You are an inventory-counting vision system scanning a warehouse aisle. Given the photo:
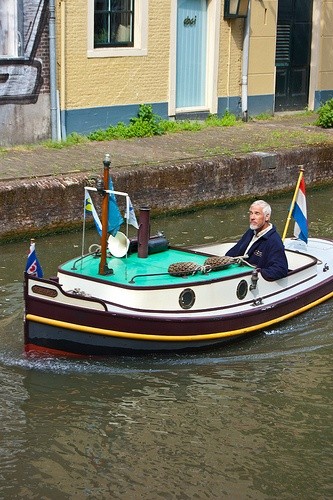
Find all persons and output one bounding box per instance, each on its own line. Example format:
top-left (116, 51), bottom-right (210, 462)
top-left (225, 200), bottom-right (288, 282)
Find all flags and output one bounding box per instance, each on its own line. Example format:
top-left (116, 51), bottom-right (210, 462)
top-left (108, 176), bottom-right (124, 237)
top-left (25, 242), bottom-right (44, 278)
top-left (85, 191), bottom-right (102, 238)
top-left (288, 174), bottom-right (308, 244)
top-left (124, 196), bottom-right (139, 229)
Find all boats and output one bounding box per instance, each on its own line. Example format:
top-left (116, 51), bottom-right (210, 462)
top-left (23, 154), bottom-right (333, 360)
